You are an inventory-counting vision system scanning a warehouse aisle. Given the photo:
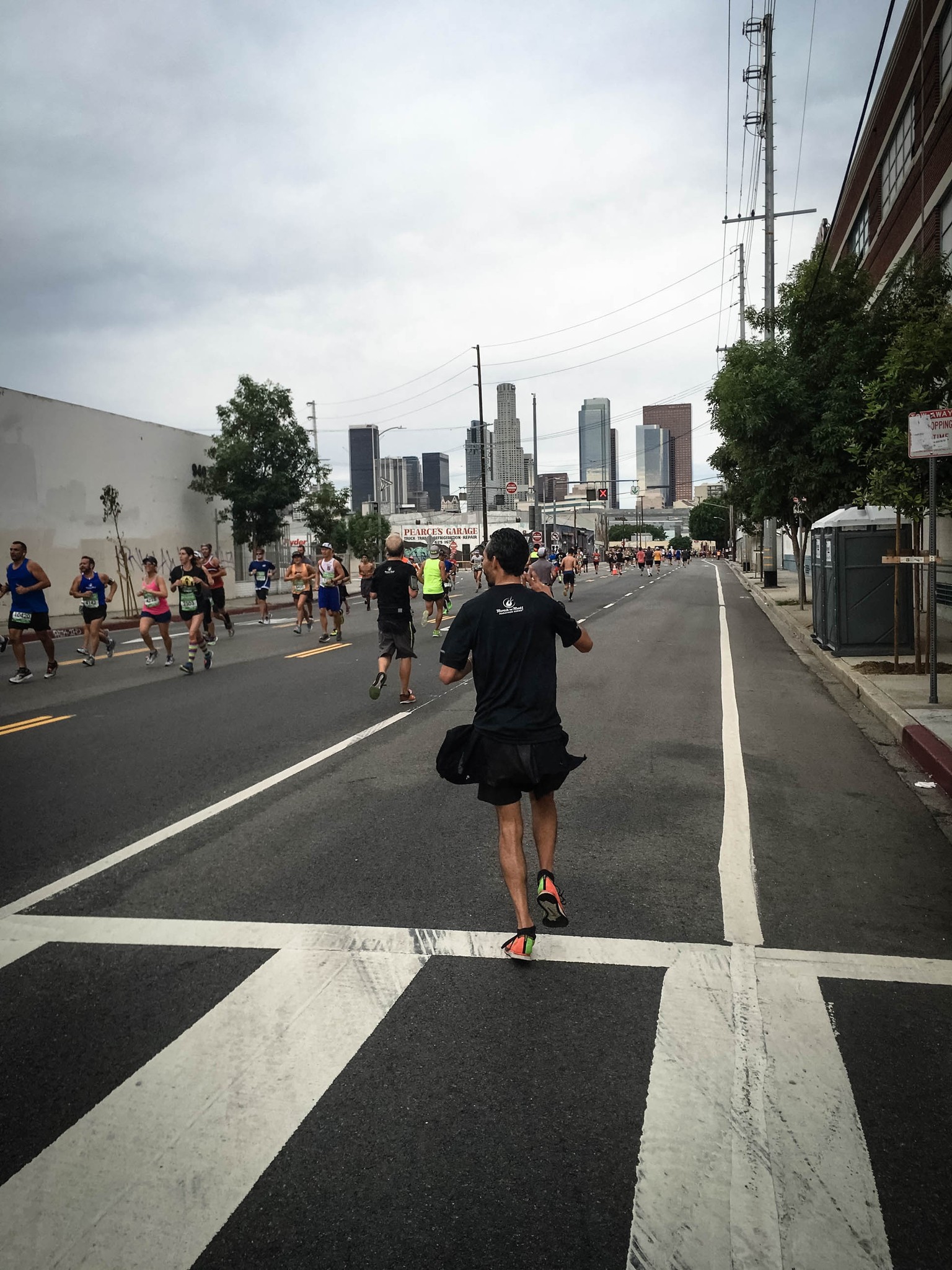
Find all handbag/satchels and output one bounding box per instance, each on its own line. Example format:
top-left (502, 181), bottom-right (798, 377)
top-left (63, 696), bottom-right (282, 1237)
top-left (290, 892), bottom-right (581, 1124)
top-left (436, 724), bottom-right (479, 784)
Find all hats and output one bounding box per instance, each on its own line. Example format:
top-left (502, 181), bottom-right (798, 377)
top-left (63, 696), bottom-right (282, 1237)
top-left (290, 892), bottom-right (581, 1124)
top-left (193, 551), bottom-right (201, 558)
top-left (474, 549), bottom-right (480, 554)
top-left (143, 555), bottom-right (157, 567)
top-left (551, 550), bottom-right (555, 551)
top-left (408, 557), bottom-right (415, 561)
top-left (537, 547), bottom-right (548, 557)
top-left (430, 545), bottom-right (446, 559)
top-left (655, 547), bottom-right (659, 550)
top-left (450, 553), bottom-right (454, 556)
top-left (292, 551), bottom-right (302, 559)
top-left (671, 546), bottom-right (673, 549)
top-left (320, 542), bottom-right (332, 550)
top-left (558, 548), bottom-right (562, 551)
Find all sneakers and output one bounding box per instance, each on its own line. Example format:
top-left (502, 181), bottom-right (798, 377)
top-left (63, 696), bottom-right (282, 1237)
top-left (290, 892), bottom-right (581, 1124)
top-left (307, 615), bottom-right (314, 632)
top-left (447, 598), bottom-right (452, 610)
top-left (329, 629), bottom-right (338, 636)
top-left (258, 618), bottom-right (264, 623)
top-left (365, 598), bottom-right (367, 604)
top-left (77, 648), bottom-right (90, 655)
top-left (228, 627), bottom-right (235, 638)
top-left (569, 598), bottom-right (573, 602)
top-left (610, 563), bottom-right (635, 577)
top-left (504, 934), bottom-right (535, 961)
top-left (563, 586), bottom-right (567, 596)
top-left (165, 653), bottom-right (175, 666)
top-left (104, 629), bottom-right (116, 658)
top-left (400, 689), bottom-right (416, 704)
top-left (663, 560), bottom-right (692, 568)
top-left (146, 648), bottom-right (159, 665)
top-left (82, 655), bottom-right (95, 666)
top-left (369, 672), bottom-right (387, 700)
top-left (179, 662), bottom-right (193, 674)
top-left (264, 613), bottom-right (272, 625)
top-left (0, 637), bottom-right (9, 653)
top-left (202, 631), bottom-right (218, 646)
top-left (640, 567), bottom-right (660, 577)
top-left (294, 625), bottom-right (302, 634)
top-left (596, 572), bottom-right (598, 574)
top-left (578, 570), bottom-right (588, 576)
top-left (421, 610), bottom-right (429, 627)
top-left (559, 580), bottom-right (562, 584)
top-left (442, 608), bottom-right (448, 615)
top-left (476, 589), bottom-right (478, 593)
top-left (556, 572), bottom-right (558, 577)
top-left (536, 875), bottom-right (569, 927)
top-left (432, 630), bottom-right (443, 637)
top-left (336, 630), bottom-right (342, 642)
top-left (479, 584), bottom-right (482, 589)
top-left (9, 667), bottom-right (33, 683)
top-left (319, 633), bottom-right (331, 643)
top-left (367, 606), bottom-right (370, 611)
top-left (339, 609), bottom-right (344, 624)
top-left (346, 605), bottom-right (350, 615)
top-left (204, 651), bottom-right (214, 669)
top-left (43, 660), bottom-right (58, 678)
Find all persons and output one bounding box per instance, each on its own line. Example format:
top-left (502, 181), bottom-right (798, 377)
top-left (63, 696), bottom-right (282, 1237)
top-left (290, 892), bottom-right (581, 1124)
top-left (437, 526), bottom-right (594, 961)
top-left (135, 555), bottom-right (176, 667)
top-left (0, 542), bottom-right (60, 685)
top-left (283, 552), bottom-right (317, 633)
top-left (192, 551), bottom-right (218, 645)
top-left (69, 556), bottom-right (118, 665)
top-left (331, 546), bottom-right (721, 638)
top-left (200, 543), bottom-right (235, 636)
top-left (367, 531), bottom-right (420, 704)
top-left (297, 544), bottom-right (316, 620)
top-left (315, 541), bottom-right (347, 645)
top-left (75, 560), bottom-right (111, 656)
top-left (248, 548), bottom-right (277, 624)
top-left (168, 547), bottom-right (214, 675)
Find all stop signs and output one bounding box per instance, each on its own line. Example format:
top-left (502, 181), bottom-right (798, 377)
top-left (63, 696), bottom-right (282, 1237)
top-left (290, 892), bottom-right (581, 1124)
top-left (505, 481), bottom-right (517, 494)
top-left (532, 532), bottom-right (542, 541)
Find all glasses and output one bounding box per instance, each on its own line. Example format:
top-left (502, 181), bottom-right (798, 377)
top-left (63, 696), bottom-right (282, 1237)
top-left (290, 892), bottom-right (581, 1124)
top-left (595, 550), bottom-right (598, 551)
top-left (256, 553), bottom-right (263, 555)
